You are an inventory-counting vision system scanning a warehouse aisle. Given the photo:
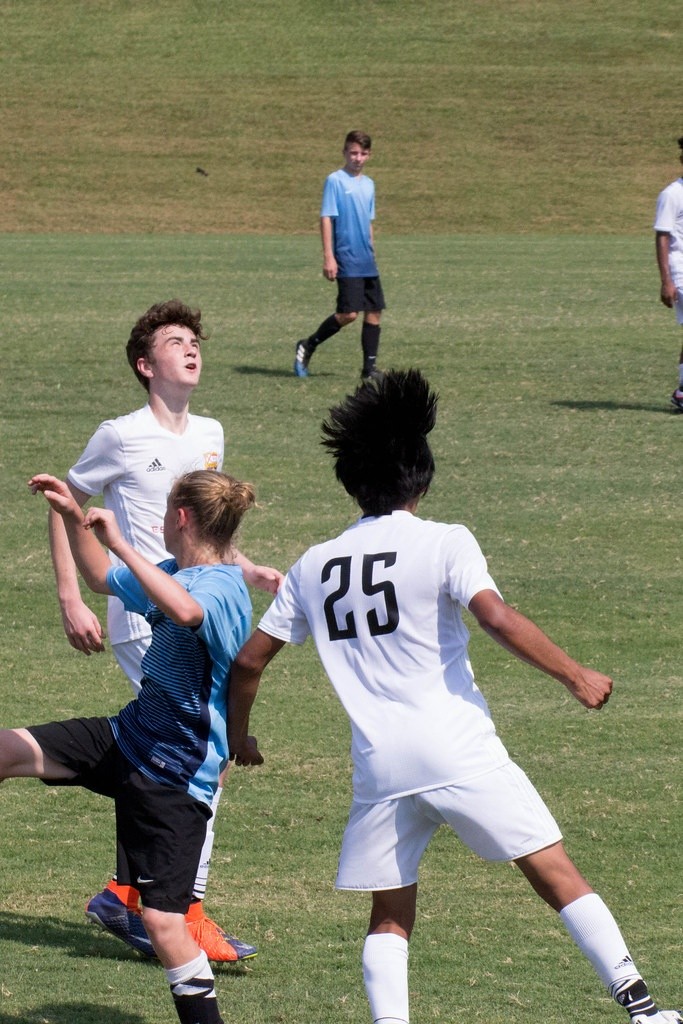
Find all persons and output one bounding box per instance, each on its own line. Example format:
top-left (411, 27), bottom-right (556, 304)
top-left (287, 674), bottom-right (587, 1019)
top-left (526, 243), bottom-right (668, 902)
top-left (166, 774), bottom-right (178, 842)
top-left (294, 130), bottom-right (387, 378)
top-left (47, 298), bottom-right (281, 967)
top-left (0, 471), bottom-right (257, 1024)
top-left (224, 369), bottom-right (683, 1024)
top-left (654, 134), bottom-right (683, 408)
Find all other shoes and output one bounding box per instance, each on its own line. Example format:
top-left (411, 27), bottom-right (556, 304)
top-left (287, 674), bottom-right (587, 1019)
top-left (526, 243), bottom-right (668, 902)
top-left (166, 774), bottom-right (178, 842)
top-left (631, 1010), bottom-right (683, 1024)
top-left (669, 387), bottom-right (683, 407)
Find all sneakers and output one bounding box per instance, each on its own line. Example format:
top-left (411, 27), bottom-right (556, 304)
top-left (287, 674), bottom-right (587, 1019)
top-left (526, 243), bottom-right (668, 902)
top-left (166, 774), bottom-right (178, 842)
top-left (361, 368), bottom-right (385, 382)
top-left (85, 888), bottom-right (158, 958)
top-left (184, 915), bottom-right (258, 967)
top-left (294, 339), bottom-right (315, 377)
